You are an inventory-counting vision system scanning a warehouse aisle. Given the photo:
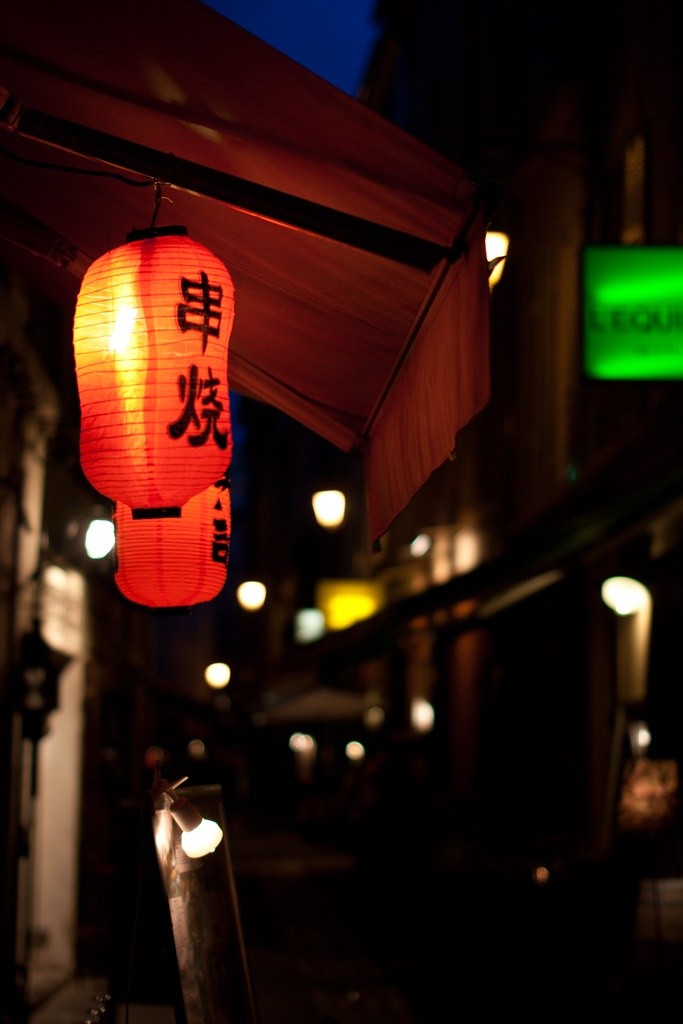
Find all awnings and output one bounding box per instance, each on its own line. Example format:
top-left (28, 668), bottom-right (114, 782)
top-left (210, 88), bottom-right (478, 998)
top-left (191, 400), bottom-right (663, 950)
top-left (0, 0), bottom-right (493, 554)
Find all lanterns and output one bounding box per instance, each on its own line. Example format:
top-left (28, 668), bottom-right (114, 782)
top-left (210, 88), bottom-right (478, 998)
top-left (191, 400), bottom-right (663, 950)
top-left (73, 225), bottom-right (233, 520)
top-left (111, 474), bottom-right (232, 608)
